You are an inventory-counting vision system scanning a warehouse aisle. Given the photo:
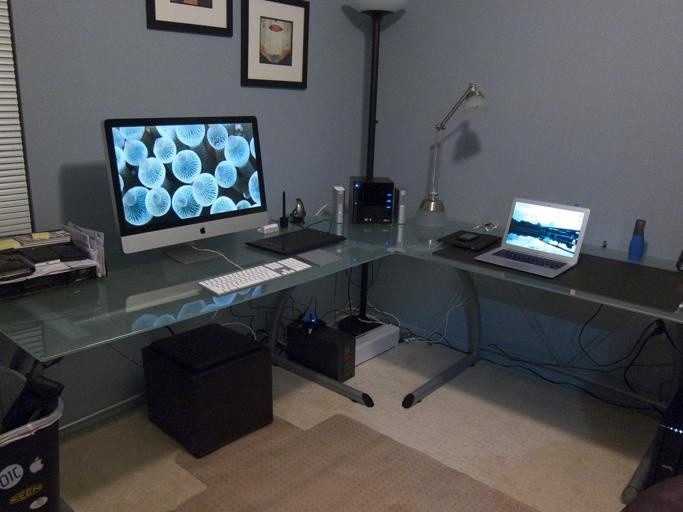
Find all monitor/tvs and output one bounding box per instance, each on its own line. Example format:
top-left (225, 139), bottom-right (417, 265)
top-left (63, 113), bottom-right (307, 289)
top-left (100, 115), bottom-right (269, 265)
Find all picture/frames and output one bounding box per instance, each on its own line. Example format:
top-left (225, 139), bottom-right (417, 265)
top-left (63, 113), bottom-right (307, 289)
top-left (145, 0), bottom-right (233, 37)
top-left (241, 0), bottom-right (309, 90)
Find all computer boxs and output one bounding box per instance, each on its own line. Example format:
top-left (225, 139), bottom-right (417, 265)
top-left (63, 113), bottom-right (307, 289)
top-left (286, 317), bottom-right (355, 383)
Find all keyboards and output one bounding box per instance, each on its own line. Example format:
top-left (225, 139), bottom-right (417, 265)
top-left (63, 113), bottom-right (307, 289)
top-left (196, 257), bottom-right (312, 296)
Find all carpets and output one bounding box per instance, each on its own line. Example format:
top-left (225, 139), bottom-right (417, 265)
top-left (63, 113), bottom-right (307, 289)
top-left (169, 413), bottom-right (539, 512)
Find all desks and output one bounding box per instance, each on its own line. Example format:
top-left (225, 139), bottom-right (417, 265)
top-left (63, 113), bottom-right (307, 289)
top-left (1, 218), bottom-right (683, 512)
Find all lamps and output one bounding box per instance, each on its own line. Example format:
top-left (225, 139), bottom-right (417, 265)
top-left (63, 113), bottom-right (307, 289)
top-left (411, 82), bottom-right (492, 227)
top-left (342, 2), bottom-right (407, 317)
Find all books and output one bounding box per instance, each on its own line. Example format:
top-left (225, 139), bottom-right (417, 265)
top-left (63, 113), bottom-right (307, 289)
top-left (0, 219), bottom-right (108, 280)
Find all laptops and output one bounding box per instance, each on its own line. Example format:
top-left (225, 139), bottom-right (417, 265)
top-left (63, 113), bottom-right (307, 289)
top-left (474, 197), bottom-right (592, 279)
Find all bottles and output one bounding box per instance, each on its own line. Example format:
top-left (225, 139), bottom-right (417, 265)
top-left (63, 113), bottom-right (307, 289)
top-left (627, 218), bottom-right (646, 259)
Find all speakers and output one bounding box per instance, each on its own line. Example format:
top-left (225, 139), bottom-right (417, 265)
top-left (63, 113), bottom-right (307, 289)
top-left (350, 176), bottom-right (396, 224)
